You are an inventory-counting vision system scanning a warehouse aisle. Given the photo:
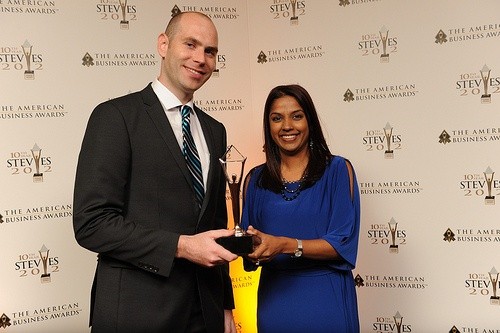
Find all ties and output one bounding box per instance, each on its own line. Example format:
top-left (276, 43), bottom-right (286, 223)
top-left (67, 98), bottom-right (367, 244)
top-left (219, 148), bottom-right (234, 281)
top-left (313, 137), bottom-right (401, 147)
top-left (178, 105), bottom-right (205, 213)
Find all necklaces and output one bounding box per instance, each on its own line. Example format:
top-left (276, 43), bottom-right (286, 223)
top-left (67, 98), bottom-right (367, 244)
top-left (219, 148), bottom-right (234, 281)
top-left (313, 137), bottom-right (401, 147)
top-left (279, 164), bottom-right (308, 200)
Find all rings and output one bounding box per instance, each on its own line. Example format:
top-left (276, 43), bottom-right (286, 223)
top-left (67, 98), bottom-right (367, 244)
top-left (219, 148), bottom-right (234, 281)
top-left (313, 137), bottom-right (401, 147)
top-left (256, 259), bottom-right (260, 266)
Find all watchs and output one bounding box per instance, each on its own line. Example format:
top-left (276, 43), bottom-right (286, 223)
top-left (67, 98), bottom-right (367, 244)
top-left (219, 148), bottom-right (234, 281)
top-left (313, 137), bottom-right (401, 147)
top-left (291, 238), bottom-right (303, 259)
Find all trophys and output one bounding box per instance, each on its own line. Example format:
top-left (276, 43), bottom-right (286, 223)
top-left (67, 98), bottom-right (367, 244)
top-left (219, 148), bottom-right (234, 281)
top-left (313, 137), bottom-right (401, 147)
top-left (219, 144), bottom-right (260, 255)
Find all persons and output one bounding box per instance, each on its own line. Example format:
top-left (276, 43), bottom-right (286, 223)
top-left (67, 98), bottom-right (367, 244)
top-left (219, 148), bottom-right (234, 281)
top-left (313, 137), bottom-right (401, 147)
top-left (73, 12), bottom-right (239, 333)
top-left (237, 85), bottom-right (361, 333)
